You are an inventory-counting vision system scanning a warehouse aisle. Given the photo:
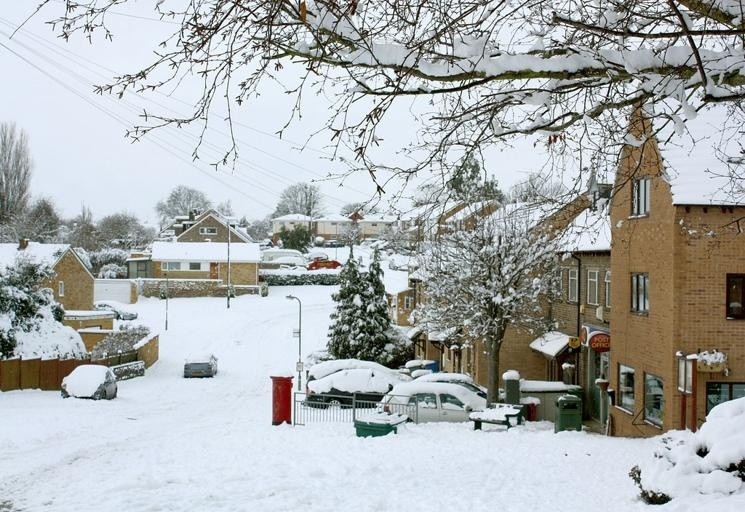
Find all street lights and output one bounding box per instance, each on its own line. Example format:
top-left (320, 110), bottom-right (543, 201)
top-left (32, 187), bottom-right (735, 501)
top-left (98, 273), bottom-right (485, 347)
top-left (287, 294), bottom-right (303, 392)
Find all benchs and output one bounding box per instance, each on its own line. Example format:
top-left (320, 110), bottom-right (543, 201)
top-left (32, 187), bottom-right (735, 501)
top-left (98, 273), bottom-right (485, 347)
top-left (468, 403), bottom-right (524, 432)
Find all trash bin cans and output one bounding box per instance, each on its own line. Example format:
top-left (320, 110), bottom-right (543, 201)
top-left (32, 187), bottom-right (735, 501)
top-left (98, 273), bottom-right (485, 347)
top-left (354, 414), bottom-right (408, 438)
top-left (556, 395), bottom-right (583, 433)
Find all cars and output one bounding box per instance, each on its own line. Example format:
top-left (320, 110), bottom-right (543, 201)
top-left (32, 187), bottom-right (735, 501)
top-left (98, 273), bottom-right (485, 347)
top-left (61, 364), bottom-right (118, 399)
top-left (94, 300), bottom-right (138, 320)
top-left (306, 358), bottom-right (487, 422)
top-left (185, 353), bottom-right (218, 378)
